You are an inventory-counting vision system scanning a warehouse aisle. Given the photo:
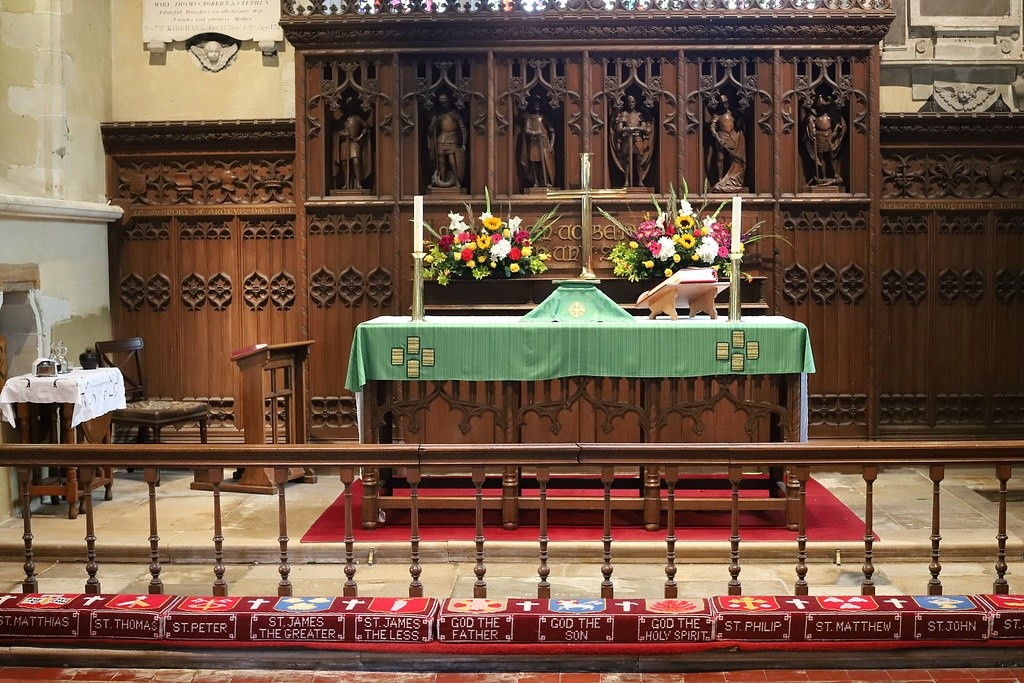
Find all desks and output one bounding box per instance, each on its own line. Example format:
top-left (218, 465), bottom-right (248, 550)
top-left (0, 366), bottom-right (128, 517)
top-left (343, 315), bottom-right (818, 532)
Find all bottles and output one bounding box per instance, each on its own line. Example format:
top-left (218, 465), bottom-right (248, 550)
top-left (47, 340), bottom-right (68, 372)
top-left (78, 346), bottom-right (99, 369)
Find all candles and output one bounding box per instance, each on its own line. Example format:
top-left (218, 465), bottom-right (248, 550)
top-left (413, 195), bottom-right (424, 253)
top-left (730, 193), bottom-right (742, 254)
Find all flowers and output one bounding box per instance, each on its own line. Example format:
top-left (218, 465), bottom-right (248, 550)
top-left (595, 177), bottom-right (797, 284)
top-left (407, 186), bottom-right (563, 286)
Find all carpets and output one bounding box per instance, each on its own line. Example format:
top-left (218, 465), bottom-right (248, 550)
top-left (299, 471), bottom-right (882, 544)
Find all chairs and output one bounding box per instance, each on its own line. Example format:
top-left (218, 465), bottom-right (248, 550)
top-left (94, 336), bottom-right (211, 487)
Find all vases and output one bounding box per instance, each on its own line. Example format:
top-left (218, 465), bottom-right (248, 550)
top-left (646, 275), bottom-right (768, 304)
top-left (531, 277), bottom-right (650, 304)
top-left (423, 277), bottom-right (531, 305)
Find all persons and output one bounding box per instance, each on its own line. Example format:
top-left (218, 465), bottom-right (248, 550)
top-left (805, 92), bottom-right (846, 186)
top-left (428, 93), bottom-right (467, 186)
top-left (956, 83), bottom-right (973, 102)
top-left (615, 95), bottom-right (652, 186)
top-left (525, 93), bottom-right (557, 188)
top-left (708, 94), bottom-right (747, 192)
top-left (332, 95), bottom-right (372, 189)
